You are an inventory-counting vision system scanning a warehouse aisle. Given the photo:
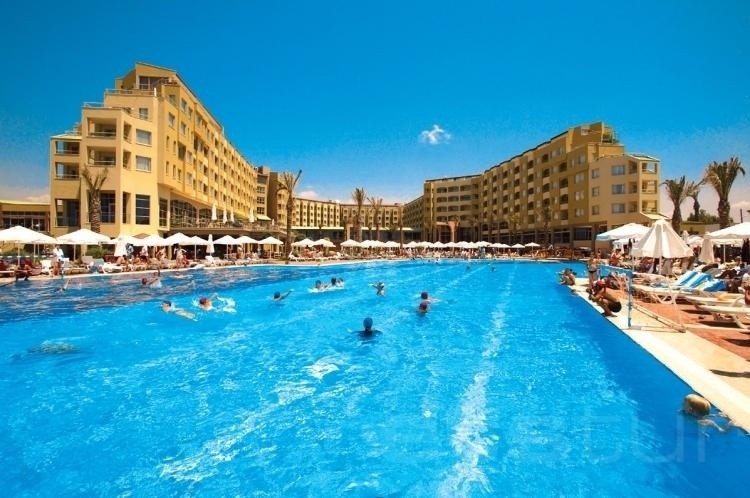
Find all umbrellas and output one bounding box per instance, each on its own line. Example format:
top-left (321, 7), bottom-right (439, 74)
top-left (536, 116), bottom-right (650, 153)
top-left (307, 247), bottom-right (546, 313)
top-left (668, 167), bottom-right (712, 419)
top-left (698, 227), bottom-right (715, 267)
top-left (595, 221), bottom-right (653, 240)
top-left (630, 216), bottom-right (698, 276)
top-left (708, 221), bottom-right (750, 244)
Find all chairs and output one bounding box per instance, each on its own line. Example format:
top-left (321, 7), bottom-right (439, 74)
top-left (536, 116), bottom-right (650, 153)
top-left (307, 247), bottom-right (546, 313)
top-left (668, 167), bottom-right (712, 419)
top-left (631, 271), bottom-right (750, 329)
top-left (182, 253), bottom-right (349, 267)
top-left (40, 253), bottom-right (188, 277)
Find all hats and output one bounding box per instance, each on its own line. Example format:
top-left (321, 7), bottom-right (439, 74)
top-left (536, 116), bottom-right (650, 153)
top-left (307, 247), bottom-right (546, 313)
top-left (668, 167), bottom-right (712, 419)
top-left (163, 299), bottom-right (171, 306)
top-left (364, 317), bottom-right (373, 327)
top-left (595, 279), bottom-right (606, 287)
top-left (316, 277), bottom-right (322, 284)
top-left (421, 291), bottom-right (427, 298)
top-left (274, 292), bottom-right (280, 298)
top-left (378, 282), bottom-right (385, 288)
top-left (200, 298), bottom-right (206, 304)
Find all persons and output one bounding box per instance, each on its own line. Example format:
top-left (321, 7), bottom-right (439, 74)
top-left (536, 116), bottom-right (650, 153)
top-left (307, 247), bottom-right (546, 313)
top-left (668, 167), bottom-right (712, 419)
top-left (569, 288), bottom-right (582, 295)
top-left (193, 290), bottom-right (219, 311)
top-left (271, 288), bottom-right (294, 302)
top-left (586, 237), bottom-right (750, 318)
top-left (555, 269), bottom-right (573, 280)
top-left (416, 300), bottom-right (429, 320)
top-left (421, 290), bottom-right (439, 305)
top-left (372, 281), bottom-right (385, 297)
top-left (159, 299), bottom-right (194, 320)
top-left (0, 244), bottom-right (196, 289)
top-left (330, 276), bottom-right (344, 287)
top-left (348, 314), bottom-right (385, 347)
top-left (677, 391), bottom-right (738, 439)
top-left (315, 280), bottom-right (328, 290)
top-left (560, 267), bottom-right (577, 286)
